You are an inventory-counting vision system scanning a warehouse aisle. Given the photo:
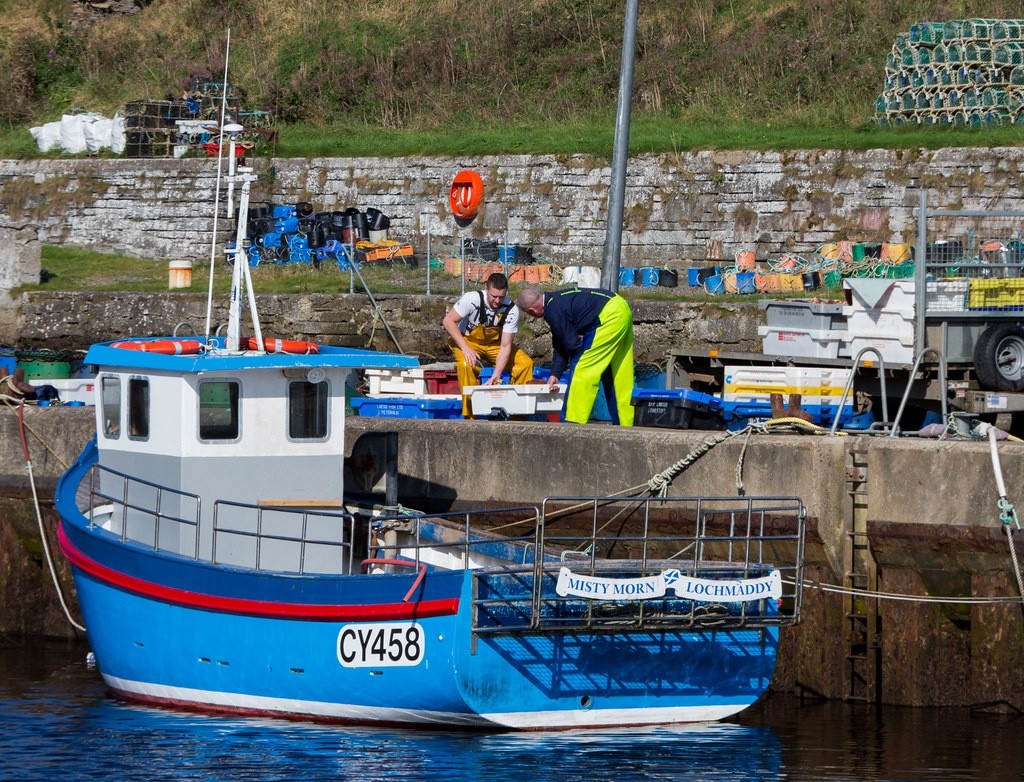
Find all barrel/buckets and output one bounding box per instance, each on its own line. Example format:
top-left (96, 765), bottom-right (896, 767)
top-left (226, 202), bottom-right (391, 272)
top-left (446, 238), bottom-right (1024, 293)
top-left (169, 260), bottom-right (193, 289)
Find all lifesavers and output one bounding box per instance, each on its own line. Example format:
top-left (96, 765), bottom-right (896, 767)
top-left (224, 335), bottom-right (321, 355)
top-left (109, 338), bottom-right (203, 356)
top-left (459, 184), bottom-right (474, 208)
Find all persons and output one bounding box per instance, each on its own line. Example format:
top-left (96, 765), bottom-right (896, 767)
top-left (444, 275), bottom-right (534, 418)
top-left (516, 288), bottom-right (638, 427)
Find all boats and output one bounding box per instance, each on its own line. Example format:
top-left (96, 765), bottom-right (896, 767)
top-left (16, 122), bottom-right (807, 733)
